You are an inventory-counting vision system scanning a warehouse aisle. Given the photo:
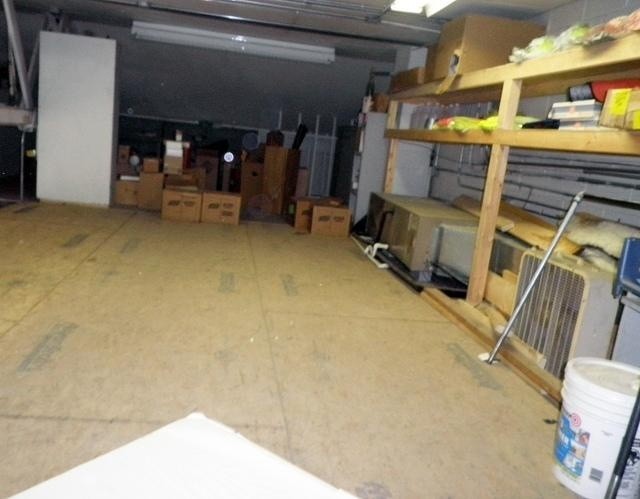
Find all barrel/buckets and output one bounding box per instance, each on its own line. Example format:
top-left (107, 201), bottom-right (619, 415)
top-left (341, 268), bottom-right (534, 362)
top-left (552, 358), bottom-right (640, 499)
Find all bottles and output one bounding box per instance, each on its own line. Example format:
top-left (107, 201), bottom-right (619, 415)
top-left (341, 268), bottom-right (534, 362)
top-left (410, 101), bottom-right (493, 132)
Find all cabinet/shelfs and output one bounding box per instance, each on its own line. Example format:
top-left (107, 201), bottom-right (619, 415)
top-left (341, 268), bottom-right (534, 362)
top-left (364, 31), bottom-right (640, 410)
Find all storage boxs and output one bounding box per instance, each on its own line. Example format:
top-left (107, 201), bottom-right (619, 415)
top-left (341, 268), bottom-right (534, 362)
top-left (114, 168), bottom-right (243, 229)
top-left (392, 13), bottom-right (545, 92)
top-left (283, 196), bottom-right (354, 238)
